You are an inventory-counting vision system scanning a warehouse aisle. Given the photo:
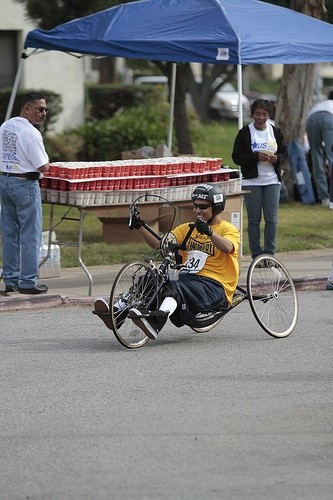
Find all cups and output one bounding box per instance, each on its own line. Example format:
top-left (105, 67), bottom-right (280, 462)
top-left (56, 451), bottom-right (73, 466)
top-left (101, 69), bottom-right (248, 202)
top-left (39, 155), bottom-right (242, 206)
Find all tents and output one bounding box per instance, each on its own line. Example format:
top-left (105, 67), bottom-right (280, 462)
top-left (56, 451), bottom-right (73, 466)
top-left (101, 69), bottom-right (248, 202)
top-left (5, 0), bottom-right (333, 157)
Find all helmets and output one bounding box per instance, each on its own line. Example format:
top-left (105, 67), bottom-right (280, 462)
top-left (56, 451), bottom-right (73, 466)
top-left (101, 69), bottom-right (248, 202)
top-left (192, 183), bottom-right (225, 214)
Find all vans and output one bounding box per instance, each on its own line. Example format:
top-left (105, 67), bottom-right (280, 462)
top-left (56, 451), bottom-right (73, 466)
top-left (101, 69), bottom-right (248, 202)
top-left (134, 76), bottom-right (252, 121)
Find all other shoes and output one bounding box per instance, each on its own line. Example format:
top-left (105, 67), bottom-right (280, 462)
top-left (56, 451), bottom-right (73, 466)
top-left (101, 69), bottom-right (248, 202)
top-left (322, 200), bottom-right (333, 209)
top-left (326, 280), bottom-right (333, 290)
top-left (255, 260), bottom-right (280, 269)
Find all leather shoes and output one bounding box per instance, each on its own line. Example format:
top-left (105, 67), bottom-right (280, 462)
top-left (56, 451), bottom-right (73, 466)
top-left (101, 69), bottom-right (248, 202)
top-left (20, 284), bottom-right (48, 295)
top-left (6, 285), bottom-right (19, 292)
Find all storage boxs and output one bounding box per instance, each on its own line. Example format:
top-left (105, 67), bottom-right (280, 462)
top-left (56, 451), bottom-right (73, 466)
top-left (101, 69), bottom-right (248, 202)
top-left (93, 194), bottom-right (242, 260)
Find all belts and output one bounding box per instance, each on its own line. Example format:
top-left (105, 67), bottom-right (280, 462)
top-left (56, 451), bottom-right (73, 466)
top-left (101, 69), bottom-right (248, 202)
top-left (4, 172), bottom-right (27, 178)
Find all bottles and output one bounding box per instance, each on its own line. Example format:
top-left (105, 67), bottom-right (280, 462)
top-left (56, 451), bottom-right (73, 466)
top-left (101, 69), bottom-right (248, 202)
top-left (38, 230), bottom-right (61, 278)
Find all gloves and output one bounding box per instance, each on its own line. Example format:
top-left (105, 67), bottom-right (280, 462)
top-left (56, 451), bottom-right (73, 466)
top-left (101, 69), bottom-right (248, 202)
top-left (196, 219), bottom-right (212, 236)
top-left (129, 209), bottom-right (142, 230)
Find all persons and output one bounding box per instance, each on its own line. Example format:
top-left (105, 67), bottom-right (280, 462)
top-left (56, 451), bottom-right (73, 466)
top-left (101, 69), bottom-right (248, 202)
top-left (231, 99), bottom-right (288, 268)
top-left (94, 183), bottom-right (242, 340)
top-left (0, 91), bottom-right (51, 293)
top-left (305, 91), bottom-right (333, 209)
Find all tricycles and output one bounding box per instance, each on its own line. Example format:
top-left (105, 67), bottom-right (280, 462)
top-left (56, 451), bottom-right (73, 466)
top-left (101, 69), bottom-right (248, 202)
top-left (91, 194), bottom-right (300, 349)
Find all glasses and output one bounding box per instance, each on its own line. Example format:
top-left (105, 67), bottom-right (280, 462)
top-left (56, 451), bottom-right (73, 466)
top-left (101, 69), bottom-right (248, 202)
top-left (27, 103), bottom-right (49, 113)
top-left (194, 203), bottom-right (211, 210)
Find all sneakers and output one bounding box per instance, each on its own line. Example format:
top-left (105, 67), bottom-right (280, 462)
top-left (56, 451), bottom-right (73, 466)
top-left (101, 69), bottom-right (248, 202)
top-left (94, 297), bottom-right (123, 331)
top-left (128, 308), bottom-right (159, 340)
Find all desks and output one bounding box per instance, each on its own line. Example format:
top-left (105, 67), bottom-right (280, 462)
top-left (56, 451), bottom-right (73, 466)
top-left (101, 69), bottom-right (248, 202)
top-left (38, 190), bottom-right (251, 296)
